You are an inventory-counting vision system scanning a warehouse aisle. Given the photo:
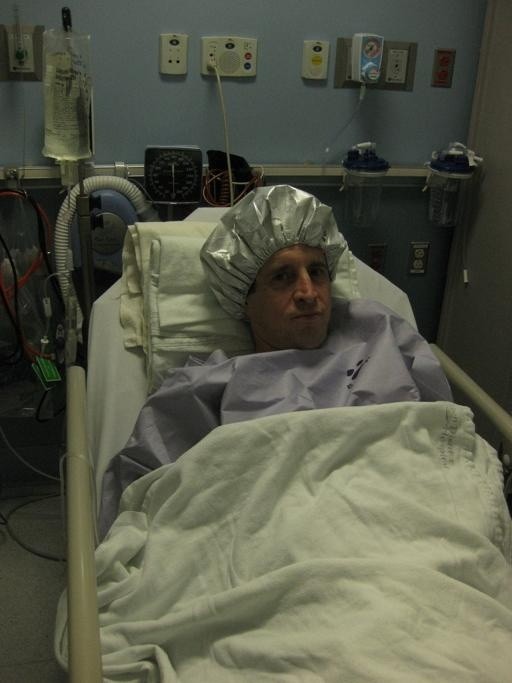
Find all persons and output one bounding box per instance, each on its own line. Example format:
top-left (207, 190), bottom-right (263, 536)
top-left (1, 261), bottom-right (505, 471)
top-left (96, 178), bottom-right (454, 539)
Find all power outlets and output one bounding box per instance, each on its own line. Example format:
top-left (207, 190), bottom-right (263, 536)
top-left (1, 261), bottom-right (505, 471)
top-left (410, 242), bottom-right (429, 274)
top-left (200, 38), bottom-right (258, 77)
top-left (8, 32), bottom-right (34, 73)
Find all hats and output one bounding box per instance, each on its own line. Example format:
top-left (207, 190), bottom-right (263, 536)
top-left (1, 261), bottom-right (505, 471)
top-left (200, 185), bottom-right (349, 322)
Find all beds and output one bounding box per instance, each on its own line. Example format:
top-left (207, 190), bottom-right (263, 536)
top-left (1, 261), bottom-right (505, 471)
top-left (65, 205), bottom-right (512, 682)
